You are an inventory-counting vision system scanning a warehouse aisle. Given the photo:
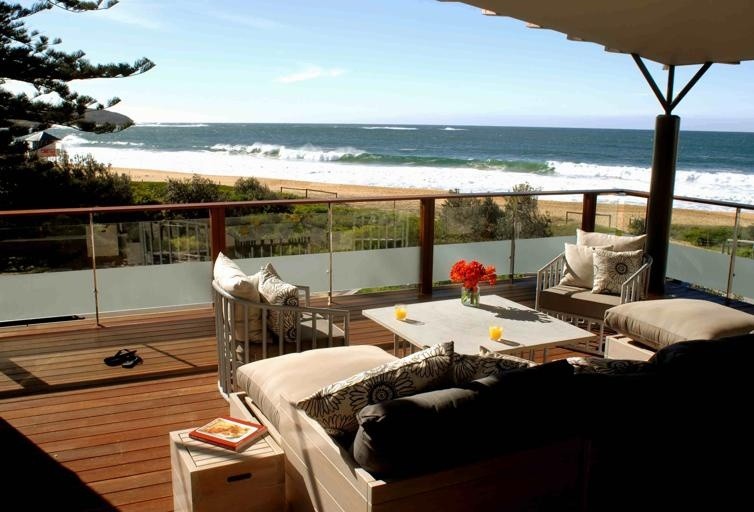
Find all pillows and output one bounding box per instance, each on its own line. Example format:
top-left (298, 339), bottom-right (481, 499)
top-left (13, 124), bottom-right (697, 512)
top-left (349, 359), bottom-right (575, 473)
top-left (592, 247), bottom-right (643, 298)
top-left (575, 227), bottom-right (649, 297)
top-left (213, 251), bottom-right (272, 344)
top-left (648, 333), bottom-right (754, 403)
top-left (603, 298), bottom-right (754, 350)
top-left (456, 352), bottom-right (530, 383)
top-left (294, 342), bottom-right (455, 479)
top-left (572, 356), bottom-right (664, 397)
top-left (479, 344), bottom-right (539, 369)
top-left (257, 263), bottom-right (298, 344)
top-left (559, 243), bottom-right (614, 291)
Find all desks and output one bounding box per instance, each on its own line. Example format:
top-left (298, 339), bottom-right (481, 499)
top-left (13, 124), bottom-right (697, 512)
top-left (361, 294), bottom-right (598, 363)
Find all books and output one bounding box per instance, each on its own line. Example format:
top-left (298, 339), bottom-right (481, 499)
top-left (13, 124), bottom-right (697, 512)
top-left (188, 414), bottom-right (269, 453)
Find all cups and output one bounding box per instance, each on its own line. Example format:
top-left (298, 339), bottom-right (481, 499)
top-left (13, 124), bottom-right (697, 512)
top-left (489, 319), bottom-right (504, 342)
top-left (395, 305), bottom-right (408, 322)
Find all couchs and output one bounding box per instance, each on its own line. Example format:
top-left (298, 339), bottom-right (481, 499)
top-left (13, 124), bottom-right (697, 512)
top-left (229, 297), bottom-right (754, 512)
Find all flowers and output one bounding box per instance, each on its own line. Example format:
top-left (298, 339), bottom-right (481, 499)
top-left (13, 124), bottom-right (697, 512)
top-left (449, 258), bottom-right (497, 308)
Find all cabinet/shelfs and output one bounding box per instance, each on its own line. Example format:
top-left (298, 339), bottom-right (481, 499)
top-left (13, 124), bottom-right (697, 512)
top-left (169, 416), bottom-right (286, 512)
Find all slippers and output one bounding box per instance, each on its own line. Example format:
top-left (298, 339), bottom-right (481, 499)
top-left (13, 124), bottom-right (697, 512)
top-left (104, 349), bottom-right (136, 366)
top-left (122, 356), bottom-right (141, 368)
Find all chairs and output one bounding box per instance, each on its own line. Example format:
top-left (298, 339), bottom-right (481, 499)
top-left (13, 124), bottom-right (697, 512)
top-left (535, 242), bottom-right (654, 338)
top-left (211, 279), bottom-right (351, 401)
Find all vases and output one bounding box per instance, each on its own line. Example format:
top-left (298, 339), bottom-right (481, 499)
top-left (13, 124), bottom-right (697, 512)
top-left (460, 286), bottom-right (481, 308)
